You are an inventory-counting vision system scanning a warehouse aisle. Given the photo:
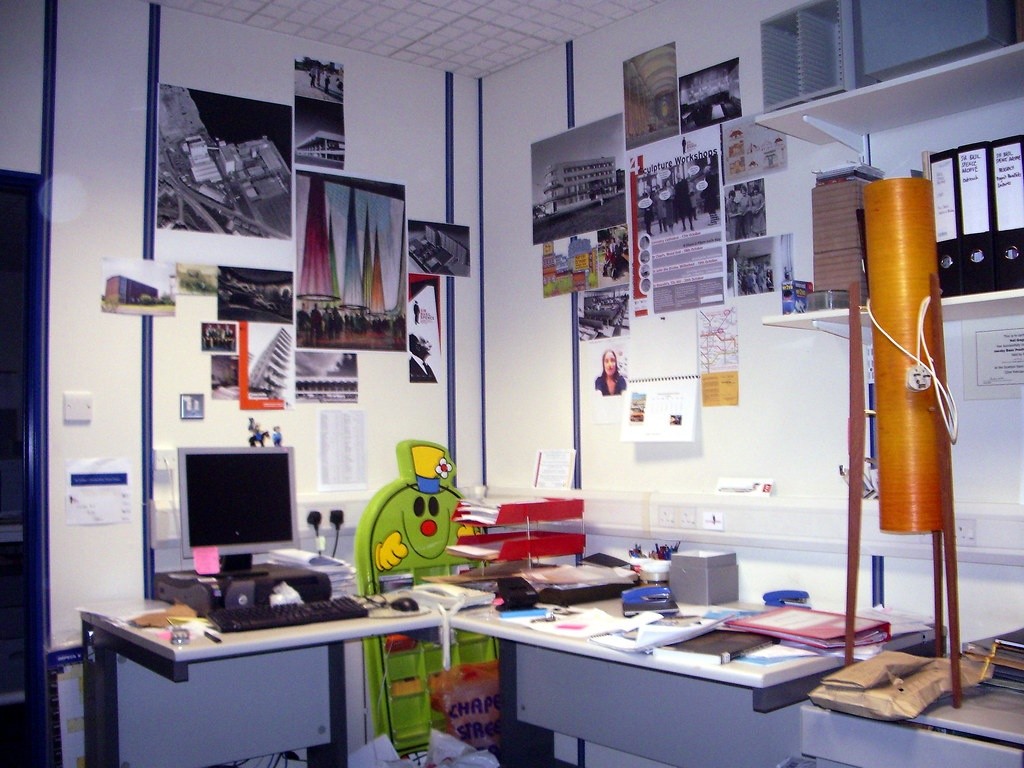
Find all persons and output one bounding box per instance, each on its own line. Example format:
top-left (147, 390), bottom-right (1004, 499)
top-left (594, 349), bottom-right (627, 396)
top-left (782, 290), bottom-right (796, 314)
top-left (738, 268), bottom-right (773, 296)
top-left (600, 232), bottom-right (629, 282)
top-left (298, 303), bottom-right (406, 347)
top-left (727, 185), bottom-right (768, 242)
top-left (309, 65), bottom-right (331, 94)
top-left (206, 324), bottom-right (233, 350)
top-left (409, 334), bottom-right (438, 382)
top-left (640, 165), bottom-right (718, 236)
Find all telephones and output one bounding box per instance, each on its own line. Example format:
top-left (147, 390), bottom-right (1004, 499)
top-left (410, 582), bottom-right (496, 611)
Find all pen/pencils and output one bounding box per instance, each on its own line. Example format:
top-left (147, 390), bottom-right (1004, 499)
top-left (204, 631), bottom-right (221, 643)
top-left (628, 543), bottom-right (644, 558)
top-left (650, 540), bottom-right (681, 560)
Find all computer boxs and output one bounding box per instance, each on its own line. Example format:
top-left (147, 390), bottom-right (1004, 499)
top-left (153, 563), bottom-right (332, 616)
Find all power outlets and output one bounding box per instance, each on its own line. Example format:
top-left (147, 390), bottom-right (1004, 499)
top-left (658, 505), bottom-right (677, 527)
top-left (956, 517), bottom-right (977, 547)
top-left (678, 507), bottom-right (697, 531)
top-left (298, 499), bottom-right (349, 532)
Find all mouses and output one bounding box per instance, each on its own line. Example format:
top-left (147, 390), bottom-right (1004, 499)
top-left (391, 597), bottom-right (419, 612)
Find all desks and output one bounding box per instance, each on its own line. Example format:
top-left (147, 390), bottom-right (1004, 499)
top-left (74, 573), bottom-right (1024, 768)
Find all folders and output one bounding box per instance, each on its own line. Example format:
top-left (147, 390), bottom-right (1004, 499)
top-left (723, 606), bottom-right (891, 649)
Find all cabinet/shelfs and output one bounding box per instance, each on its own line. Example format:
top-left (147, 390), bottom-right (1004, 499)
top-left (755, 0), bottom-right (1024, 346)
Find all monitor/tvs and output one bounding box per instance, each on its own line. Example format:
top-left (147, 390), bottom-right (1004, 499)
top-left (178, 446), bottom-right (300, 580)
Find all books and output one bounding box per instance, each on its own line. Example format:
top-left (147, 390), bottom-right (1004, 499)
top-left (410, 583), bottom-right (496, 610)
top-left (589, 615), bottom-right (780, 665)
top-left (961, 627), bottom-right (1024, 692)
top-left (814, 162), bottom-right (885, 186)
top-left (921, 151), bottom-right (938, 180)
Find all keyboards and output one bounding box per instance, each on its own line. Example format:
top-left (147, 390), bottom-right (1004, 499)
top-left (205, 596), bottom-right (369, 633)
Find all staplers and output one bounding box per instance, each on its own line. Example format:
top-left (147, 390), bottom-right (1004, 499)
top-left (621, 585), bottom-right (680, 617)
top-left (763, 590), bottom-right (812, 609)
